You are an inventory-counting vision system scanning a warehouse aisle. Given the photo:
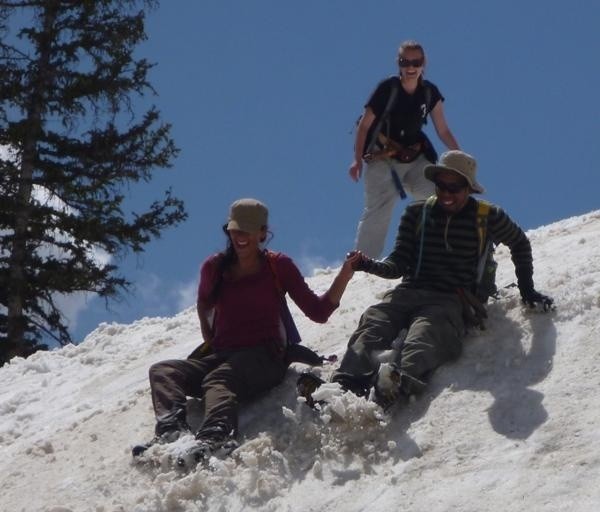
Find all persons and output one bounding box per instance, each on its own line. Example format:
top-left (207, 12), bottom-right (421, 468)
top-left (129, 197), bottom-right (361, 469)
top-left (349, 43), bottom-right (462, 260)
top-left (297, 149), bottom-right (558, 420)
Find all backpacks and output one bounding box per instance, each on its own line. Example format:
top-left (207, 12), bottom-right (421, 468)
top-left (416, 195), bottom-right (497, 303)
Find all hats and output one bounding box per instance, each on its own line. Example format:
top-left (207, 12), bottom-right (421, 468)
top-left (424, 150), bottom-right (484, 194)
top-left (227, 199), bottom-right (268, 234)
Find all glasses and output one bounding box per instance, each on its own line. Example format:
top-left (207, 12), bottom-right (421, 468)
top-left (399, 56), bottom-right (424, 67)
top-left (438, 181), bottom-right (457, 193)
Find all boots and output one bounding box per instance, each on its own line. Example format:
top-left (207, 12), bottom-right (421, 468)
top-left (372, 373), bottom-right (427, 414)
top-left (296, 372), bottom-right (365, 411)
top-left (132, 407), bottom-right (239, 467)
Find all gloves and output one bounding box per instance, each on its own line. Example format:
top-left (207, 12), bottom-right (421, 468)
top-left (519, 289), bottom-right (553, 312)
